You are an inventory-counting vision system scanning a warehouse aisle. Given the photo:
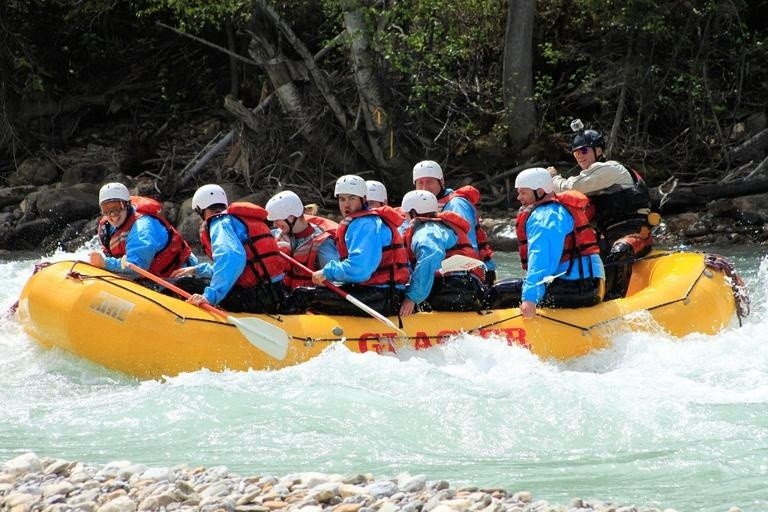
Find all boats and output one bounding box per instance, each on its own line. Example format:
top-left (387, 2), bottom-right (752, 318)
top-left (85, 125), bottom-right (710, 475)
top-left (18, 250), bottom-right (754, 387)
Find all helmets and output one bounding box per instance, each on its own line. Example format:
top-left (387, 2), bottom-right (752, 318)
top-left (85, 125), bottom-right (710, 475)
top-left (412, 160), bottom-right (445, 186)
top-left (365, 180), bottom-right (388, 204)
top-left (571, 130), bottom-right (605, 151)
top-left (265, 190), bottom-right (304, 221)
top-left (400, 190), bottom-right (438, 214)
top-left (334, 174), bottom-right (367, 199)
top-left (99, 182), bottom-right (132, 205)
top-left (192, 184), bottom-right (228, 210)
top-left (515, 168), bottom-right (554, 195)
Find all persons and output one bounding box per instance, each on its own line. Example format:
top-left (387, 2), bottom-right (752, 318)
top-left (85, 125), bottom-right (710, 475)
top-left (548, 118), bottom-right (652, 301)
top-left (413, 159), bottom-right (496, 286)
top-left (398, 190), bottom-right (484, 316)
top-left (265, 189), bottom-right (341, 290)
top-left (90, 182), bottom-right (199, 292)
top-left (365, 179), bottom-right (410, 237)
top-left (161, 184), bottom-right (286, 314)
top-left (284, 173), bottom-right (409, 317)
top-left (484, 167), bottom-right (607, 320)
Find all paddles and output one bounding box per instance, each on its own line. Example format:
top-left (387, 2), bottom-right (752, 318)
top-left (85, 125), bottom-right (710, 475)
top-left (279, 246), bottom-right (407, 339)
top-left (121, 255), bottom-right (288, 360)
